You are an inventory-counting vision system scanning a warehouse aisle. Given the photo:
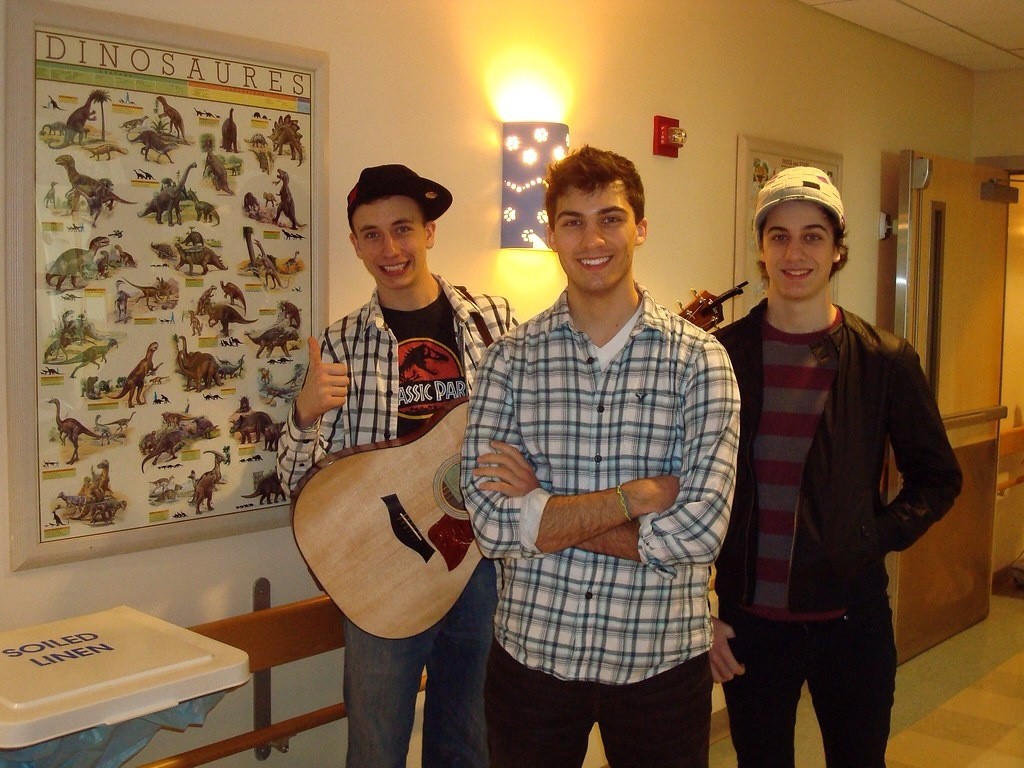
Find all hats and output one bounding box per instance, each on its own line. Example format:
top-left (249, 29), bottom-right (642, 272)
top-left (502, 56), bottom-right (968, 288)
top-left (750, 164), bottom-right (846, 243)
top-left (345, 162), bottom-right (455, 224)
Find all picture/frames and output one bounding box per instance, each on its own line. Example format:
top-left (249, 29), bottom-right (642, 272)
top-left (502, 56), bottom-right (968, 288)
top-left (732, 134), bottom-right (846, 327)
top-left (0, 0), bottom-right (329, 573)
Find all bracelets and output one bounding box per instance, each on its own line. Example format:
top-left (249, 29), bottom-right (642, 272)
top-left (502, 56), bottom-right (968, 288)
top-left (616, 484), bottom-right (633, 522)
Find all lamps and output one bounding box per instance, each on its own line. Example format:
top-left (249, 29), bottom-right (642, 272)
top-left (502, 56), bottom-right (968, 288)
top-left (501, 121), bottom-right (570, 249)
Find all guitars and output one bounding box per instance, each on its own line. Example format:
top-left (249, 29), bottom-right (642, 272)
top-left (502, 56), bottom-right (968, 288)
top-left (292, 278), bottom-right (750, 641)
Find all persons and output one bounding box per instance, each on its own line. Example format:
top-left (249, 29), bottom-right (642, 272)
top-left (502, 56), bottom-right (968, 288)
top-left (276, 163), bottom-right (520, 768)
top-left (461, 144), bottom-right (741, 768)
top-left (711, 166), bottom-right (964, 768)
